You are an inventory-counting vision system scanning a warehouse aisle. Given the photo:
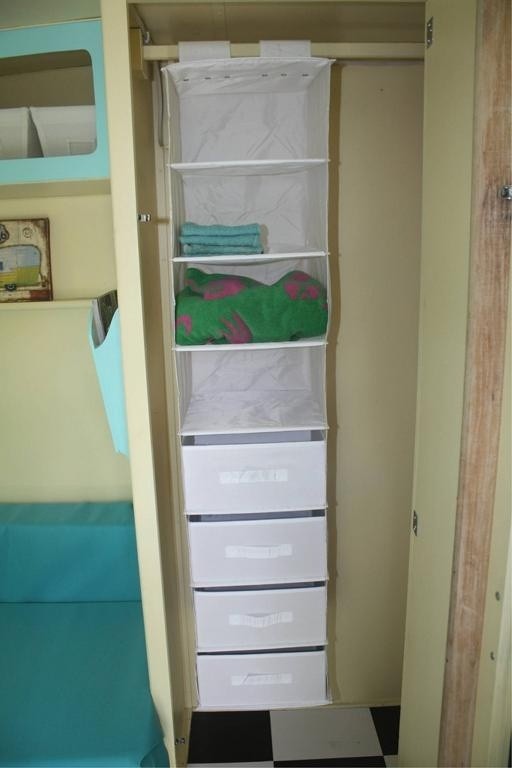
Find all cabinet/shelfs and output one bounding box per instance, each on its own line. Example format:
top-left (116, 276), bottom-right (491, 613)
top-left (160, 40), bottom-right (330, 714)
top-left (0, 18), bottom-right (114, 312)
